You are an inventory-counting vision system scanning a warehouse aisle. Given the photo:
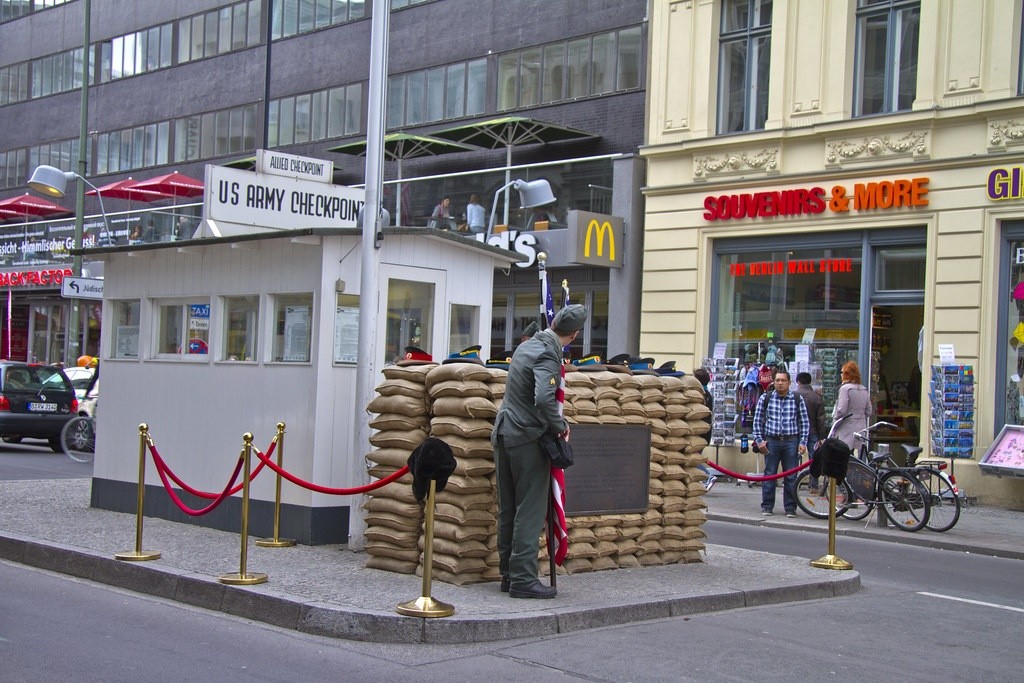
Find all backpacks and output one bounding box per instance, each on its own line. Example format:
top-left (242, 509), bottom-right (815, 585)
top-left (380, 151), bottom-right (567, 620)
top-left (809, 437), bottom-right (850, 486)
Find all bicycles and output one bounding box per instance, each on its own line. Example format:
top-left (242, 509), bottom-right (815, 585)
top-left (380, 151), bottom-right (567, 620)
top-left (823, 421), bottom-right (961, 533)
top-left (795, 413), bottom-right (929, 532)
top-left (60, 395), bottom-right (95, 464)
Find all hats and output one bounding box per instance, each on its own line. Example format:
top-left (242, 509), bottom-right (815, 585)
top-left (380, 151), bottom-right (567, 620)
top-left (554, 304), bottom-right (587, 332)
top-left (604, 354), bottom-right (676, 373)
top-left (737, 365), bottom-right (774, 412)
top-left (578, 351), bottom-right (602, 363)
top-left (403, 346), bottom-right (433, 362)
top-left (460, 345), bottom-right (481, 356)
top-left (521, 321), bottom-right (541, 336)
top-left (486, 351), bottom-right (514, 363)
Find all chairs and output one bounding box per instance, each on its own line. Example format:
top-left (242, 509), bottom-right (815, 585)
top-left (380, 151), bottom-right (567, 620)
top-left (8, 372), bottom-right (24, 384)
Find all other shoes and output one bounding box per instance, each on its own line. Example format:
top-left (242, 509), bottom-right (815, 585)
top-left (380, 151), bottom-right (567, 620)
top-left (785, 511), bottom-right (797, 517)
top-left (761, 507), bottom-right (773, 515)
top-left (810, 487), bottom-right (818, 494)
top-left (500, 575), bottom-right (557, 599)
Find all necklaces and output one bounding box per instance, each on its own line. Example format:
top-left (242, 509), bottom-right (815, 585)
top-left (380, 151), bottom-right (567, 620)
top-left (9, 372), bottom-right (30, 386)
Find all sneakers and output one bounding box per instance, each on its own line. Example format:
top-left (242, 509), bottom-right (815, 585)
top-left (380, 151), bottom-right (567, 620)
top-left (705, 475), bottom-right (717, 491)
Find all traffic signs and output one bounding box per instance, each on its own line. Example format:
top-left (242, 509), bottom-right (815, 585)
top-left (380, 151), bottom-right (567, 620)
top-left (60, 277), bottom-right (103, 301)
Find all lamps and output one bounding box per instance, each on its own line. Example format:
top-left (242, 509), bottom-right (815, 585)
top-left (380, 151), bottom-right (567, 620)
top-left (487, 180), bottom-right (556, 243)
top-left (27, 165), bottom-right (112, 246)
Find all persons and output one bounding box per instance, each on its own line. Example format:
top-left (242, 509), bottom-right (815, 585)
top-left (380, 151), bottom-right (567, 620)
top-left (429, 195), bottom-right (457, 230)
top-left (692, 369), bottom-right (718, 491)
top-left (794, 372), bottom-right (826, 494)
top-left (465, 192), bottom-right (486, 231)
top-left (831, 359), bottom-right (872, 508)
top-left (129, 223), bottom-right (142, 241)
top-left (489, 302), bottom-right (588, 598)
top-left (520, 320), bottom-right (541, 341)
top-left (176, 216), bottom-right (194, 240)
top-left (143, 219), bottom-right (159, 241)
top-left (752, 369), bottom-right (809, 517)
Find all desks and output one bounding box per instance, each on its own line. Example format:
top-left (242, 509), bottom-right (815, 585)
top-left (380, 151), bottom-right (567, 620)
top-left (416, 216), bottom-right (463, 231)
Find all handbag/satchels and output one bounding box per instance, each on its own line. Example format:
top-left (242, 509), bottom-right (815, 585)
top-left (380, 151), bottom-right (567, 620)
top-left (751, 433), bottom-right (766, 453)
top-left (541, 426), bottom-right (574, 469)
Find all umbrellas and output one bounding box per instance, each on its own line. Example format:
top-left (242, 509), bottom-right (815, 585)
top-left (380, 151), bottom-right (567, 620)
top-left (0, 191), bottom-right (69, 261)
top-left (83, 155), bottom-right (257, 244)
top-left (321, 128), bottom-right (475, 226)
top-left (427, 113), bottom-right (600, 228)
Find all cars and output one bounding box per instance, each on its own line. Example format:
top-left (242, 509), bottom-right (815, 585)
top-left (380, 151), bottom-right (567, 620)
top-left (42, 367), bottom-right (99, 452)
top-left (0, 361), bottom-right (80, 453)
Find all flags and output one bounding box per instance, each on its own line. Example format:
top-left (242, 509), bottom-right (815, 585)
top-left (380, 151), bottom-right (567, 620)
top-left (538, 269), bottom-right (577, 567)
